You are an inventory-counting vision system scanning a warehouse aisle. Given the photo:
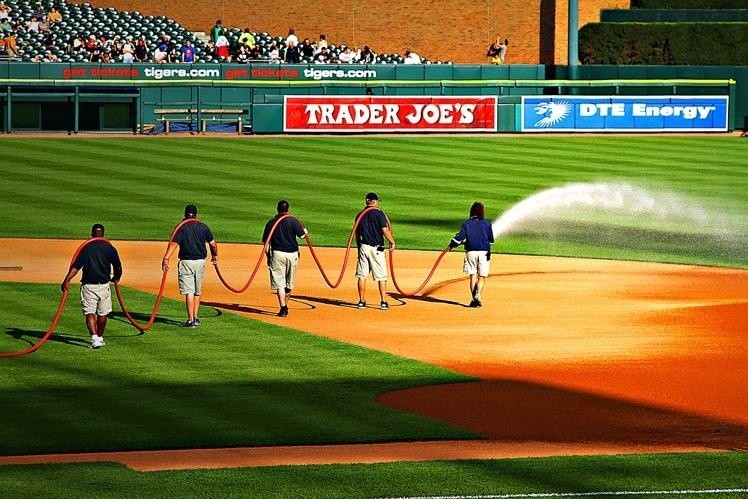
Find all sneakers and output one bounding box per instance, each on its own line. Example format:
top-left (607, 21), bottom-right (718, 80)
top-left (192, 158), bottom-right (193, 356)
top-left (88, 339), bottom-right (101, 349)
top-left (381, 302), bottom-right (390, 310)
top-left (277, 308), bottom-right (288, 316)
top-left (99, 338), bottom-right (105, 346)
top-left (473, 294), bottom-right (482, 306)
top-left (182, 321), bottom-right (195, 329)
top-left (469, 300), bottom-right (476, 306)
top-left (194, 319), bottom-right (200, 326)
top-left (358, 300), bottom-right (369, 308)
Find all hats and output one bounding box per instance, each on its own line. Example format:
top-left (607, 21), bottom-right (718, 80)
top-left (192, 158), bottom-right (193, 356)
top-left (365, 192), bottom-right (381, 200)
top-left (92, 224), bottom-right (104, 232)
top-left (184, 205), bottom-right (197, 214)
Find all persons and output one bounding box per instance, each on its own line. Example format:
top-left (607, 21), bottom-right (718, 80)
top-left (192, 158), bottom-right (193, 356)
top-left (73, 34), bottom-right (148, 64)
top-left (155, 34), bottom-right (196, 63)
top-left (60, 222), bottom-right (123, 347)
top-left (352, 192), bottom-right (397, 312)
top-left (491, 53), bottom-right (503, 65)
top-left (269, 30), bottom-right (353, 65)
top-left (443, 199), bottom-right (496, 310)
top-left (404, 51), bottom-right (421, 65)
top-left (31, 54), bottom-right (61, 63)
top-left (261, 200), bottom-right (309, 318)
top-left (364, 87), bottom-right (374, 94)
top-left (1, 1), bottom-right (21, 57)
top-left (160, 205), bottom-right (220, 332)
top-left (211, 19), bottom-right (261, 64)
top-left (25, 5), bottom-right (62, 33)
top-left (495, 36), bottom-right (509, 64)
top-left (360, 45), bottom-right (374, 64)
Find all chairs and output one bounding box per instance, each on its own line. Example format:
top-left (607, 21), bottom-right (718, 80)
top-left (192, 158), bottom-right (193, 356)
top-left (0, 0), bottom-right (453, 64)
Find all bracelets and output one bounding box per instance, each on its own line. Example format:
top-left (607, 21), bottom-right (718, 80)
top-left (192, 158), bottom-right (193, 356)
top-left (163, 256), bottom-right (169, 260)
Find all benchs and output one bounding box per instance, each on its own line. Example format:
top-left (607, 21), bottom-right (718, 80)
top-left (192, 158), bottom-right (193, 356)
top-left (153, 108), bottom-right (244, 135)
